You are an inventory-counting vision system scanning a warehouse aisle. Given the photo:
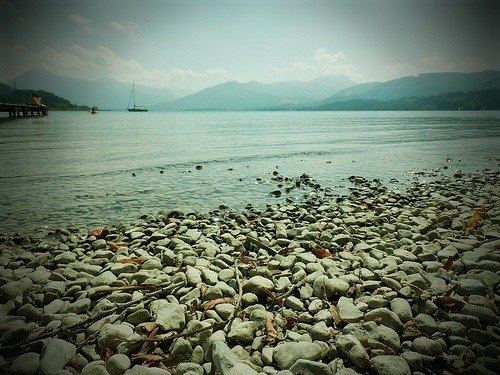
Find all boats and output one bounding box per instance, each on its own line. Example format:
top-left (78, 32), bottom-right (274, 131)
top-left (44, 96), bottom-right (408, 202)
top-left (88, 109), bottom-right (99, 115)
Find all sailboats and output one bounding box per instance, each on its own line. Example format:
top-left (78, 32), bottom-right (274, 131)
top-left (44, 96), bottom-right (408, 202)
top-left (127, 79), bottom-right (148, 112)
